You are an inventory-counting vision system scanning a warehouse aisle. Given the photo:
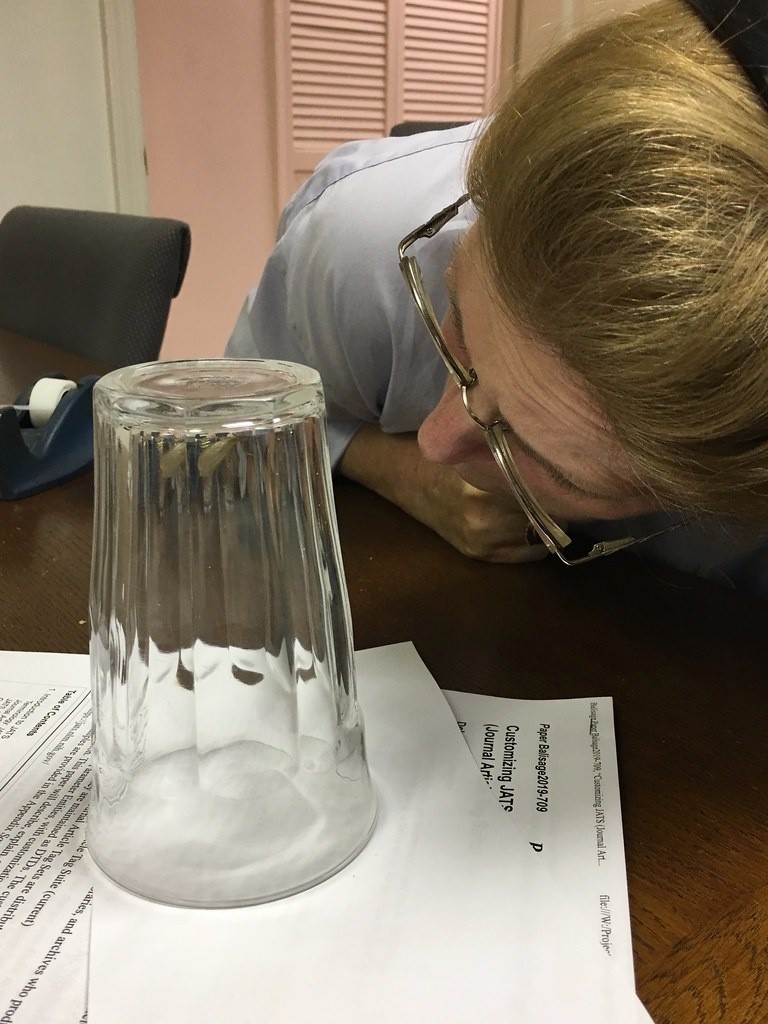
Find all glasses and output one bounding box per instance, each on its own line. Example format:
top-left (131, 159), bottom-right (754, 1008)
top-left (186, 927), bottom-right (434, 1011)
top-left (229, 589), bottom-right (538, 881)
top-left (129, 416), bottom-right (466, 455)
top-left (397, 188), bottom-right (708, 569)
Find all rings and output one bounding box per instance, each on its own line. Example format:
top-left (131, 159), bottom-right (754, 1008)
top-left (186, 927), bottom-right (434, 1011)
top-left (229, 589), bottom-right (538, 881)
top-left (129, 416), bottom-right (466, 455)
top-left (525, 523), bottom-right (542, 547)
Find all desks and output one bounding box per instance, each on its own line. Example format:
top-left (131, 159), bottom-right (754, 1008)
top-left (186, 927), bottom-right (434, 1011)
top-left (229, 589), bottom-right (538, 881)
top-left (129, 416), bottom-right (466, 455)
top-left (0, 469), bottom-right (768, 1024)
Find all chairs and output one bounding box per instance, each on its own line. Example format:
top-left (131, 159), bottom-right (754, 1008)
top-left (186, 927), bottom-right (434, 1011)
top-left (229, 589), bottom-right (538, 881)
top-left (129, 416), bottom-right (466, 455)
top-left (0, 205), bottom-right (192, 406)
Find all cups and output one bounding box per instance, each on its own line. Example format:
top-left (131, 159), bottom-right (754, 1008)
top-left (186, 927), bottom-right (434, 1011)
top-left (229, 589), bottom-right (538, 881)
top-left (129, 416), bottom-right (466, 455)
top-left (88, 359), bottom-right (379, 907)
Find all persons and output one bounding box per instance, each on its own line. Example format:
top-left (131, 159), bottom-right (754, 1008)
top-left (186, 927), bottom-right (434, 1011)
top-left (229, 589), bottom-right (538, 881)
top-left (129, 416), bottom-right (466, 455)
top-left (221, 0), bottom-right (768, 564)
